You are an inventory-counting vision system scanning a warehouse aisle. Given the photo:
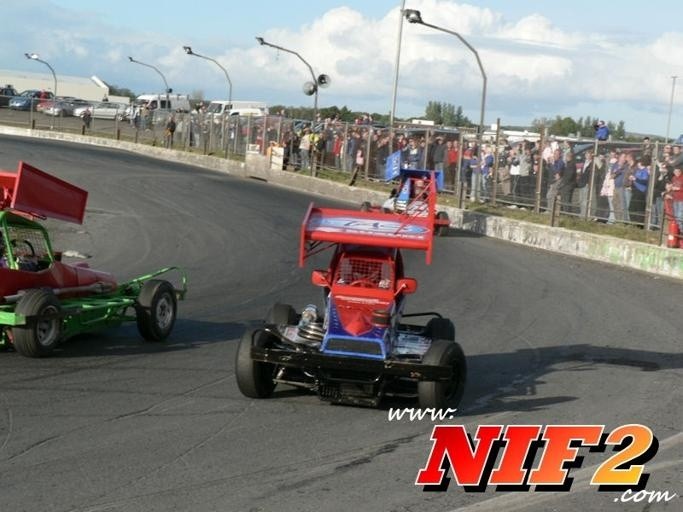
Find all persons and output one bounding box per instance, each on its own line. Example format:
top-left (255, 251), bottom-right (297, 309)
top-left (78, 93), bottom-right (683, 235)
top-left (0, 230), bottom-right (41, 272)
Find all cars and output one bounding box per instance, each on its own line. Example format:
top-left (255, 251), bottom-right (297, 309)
top-left (0, 86), bottom-right (682, 202)
top-left (356, 150), bottom-right (449, 238)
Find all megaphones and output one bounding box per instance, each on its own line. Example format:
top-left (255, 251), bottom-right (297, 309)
top-left (303, 81), bottom-right (316, 96)
top-left (317, 73), bottom-right (331, 89)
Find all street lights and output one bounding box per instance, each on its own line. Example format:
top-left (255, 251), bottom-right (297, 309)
top-left (253, 34), bottom-right (318, 177)
top-left (182, 43), bottom-right (233, 115)
top-left (127, 54), bottom-right (169, 111)
top-left (403, 8), bottom-right (486, 204)
top-left (23, 51), bottom-right (58, 126)
